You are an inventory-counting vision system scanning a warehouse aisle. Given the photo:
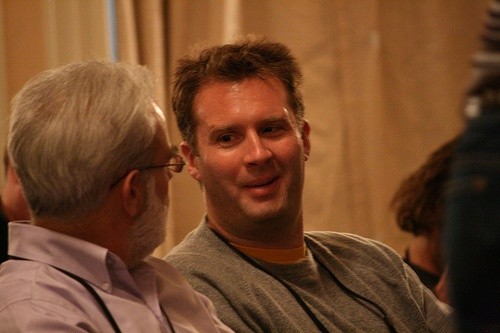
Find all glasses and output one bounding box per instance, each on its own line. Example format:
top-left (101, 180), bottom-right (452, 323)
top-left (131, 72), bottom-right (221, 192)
top-left (110, 154), bottom-right (185, 191)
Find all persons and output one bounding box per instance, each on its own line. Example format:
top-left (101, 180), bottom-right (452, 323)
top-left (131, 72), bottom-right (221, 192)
top-left (390, 137), bottom-right (454, 290)
top-left (0, 145), bottom-right (30, 263)
top-left (163, 39), bottom-right (451, 333)
top-left (0, 57), bottom-right (234, 332)
